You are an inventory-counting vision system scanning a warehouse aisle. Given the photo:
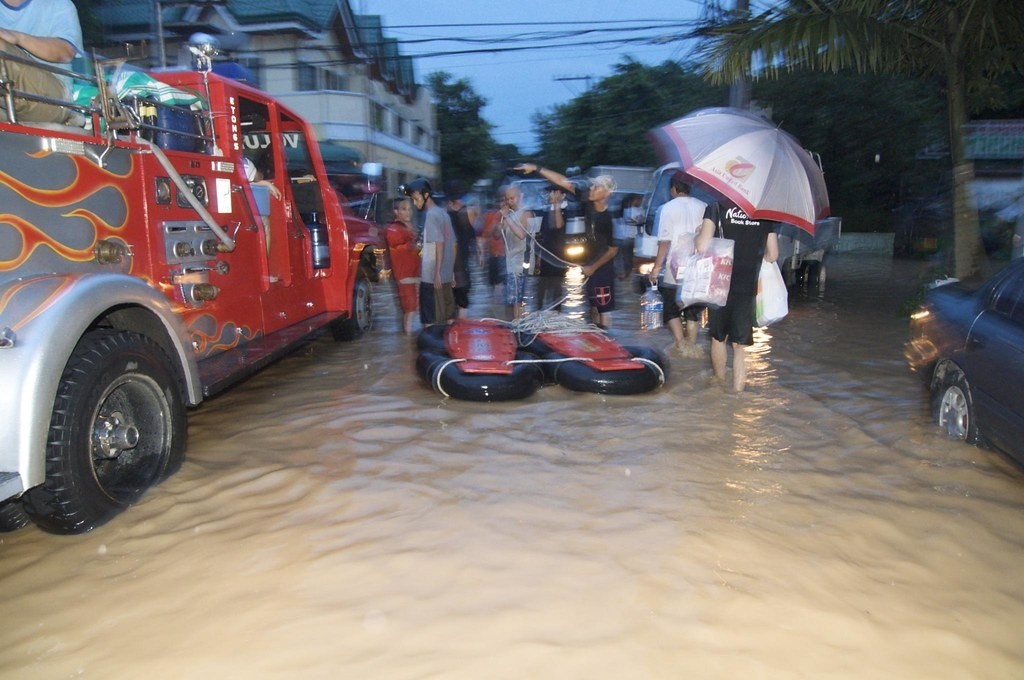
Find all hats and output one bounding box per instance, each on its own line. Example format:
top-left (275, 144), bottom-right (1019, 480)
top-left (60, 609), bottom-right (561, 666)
top-left (544, 184), bottom-right (568, 194)
top-left (589, 174), bottom-right (617, 196)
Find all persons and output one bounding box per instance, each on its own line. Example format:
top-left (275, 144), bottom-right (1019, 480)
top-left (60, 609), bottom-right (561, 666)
top-left (241, 141), bottom-right (1024, 390)
top-left (0, 0), bottom-right (83, 123)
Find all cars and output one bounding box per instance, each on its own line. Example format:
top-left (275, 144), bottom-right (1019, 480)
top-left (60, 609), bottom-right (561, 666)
top-left (510, 162), bottom-right (724, 292)
top-left (906, 253), bottom-right (1024, 473)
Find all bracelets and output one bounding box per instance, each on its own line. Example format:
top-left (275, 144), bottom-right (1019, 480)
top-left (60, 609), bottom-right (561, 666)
top-left (534, 165), bottom-right (543, 175)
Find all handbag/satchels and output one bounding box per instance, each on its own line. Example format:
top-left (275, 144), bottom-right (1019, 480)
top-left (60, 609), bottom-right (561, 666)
top-left (751, 258), bottom-right (789, 328)
top-left (675, 202), bottom-right (735, 310)
top-left (670, 232), bottom-right (700, 283)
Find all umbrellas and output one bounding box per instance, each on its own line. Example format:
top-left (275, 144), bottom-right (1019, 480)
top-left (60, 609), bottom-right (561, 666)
top-left (662, 106), bottom-right (834, 236)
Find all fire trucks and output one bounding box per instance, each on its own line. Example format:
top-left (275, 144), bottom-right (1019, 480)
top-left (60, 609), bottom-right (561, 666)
top-left (0, 37), bottom-right (391, 535)
